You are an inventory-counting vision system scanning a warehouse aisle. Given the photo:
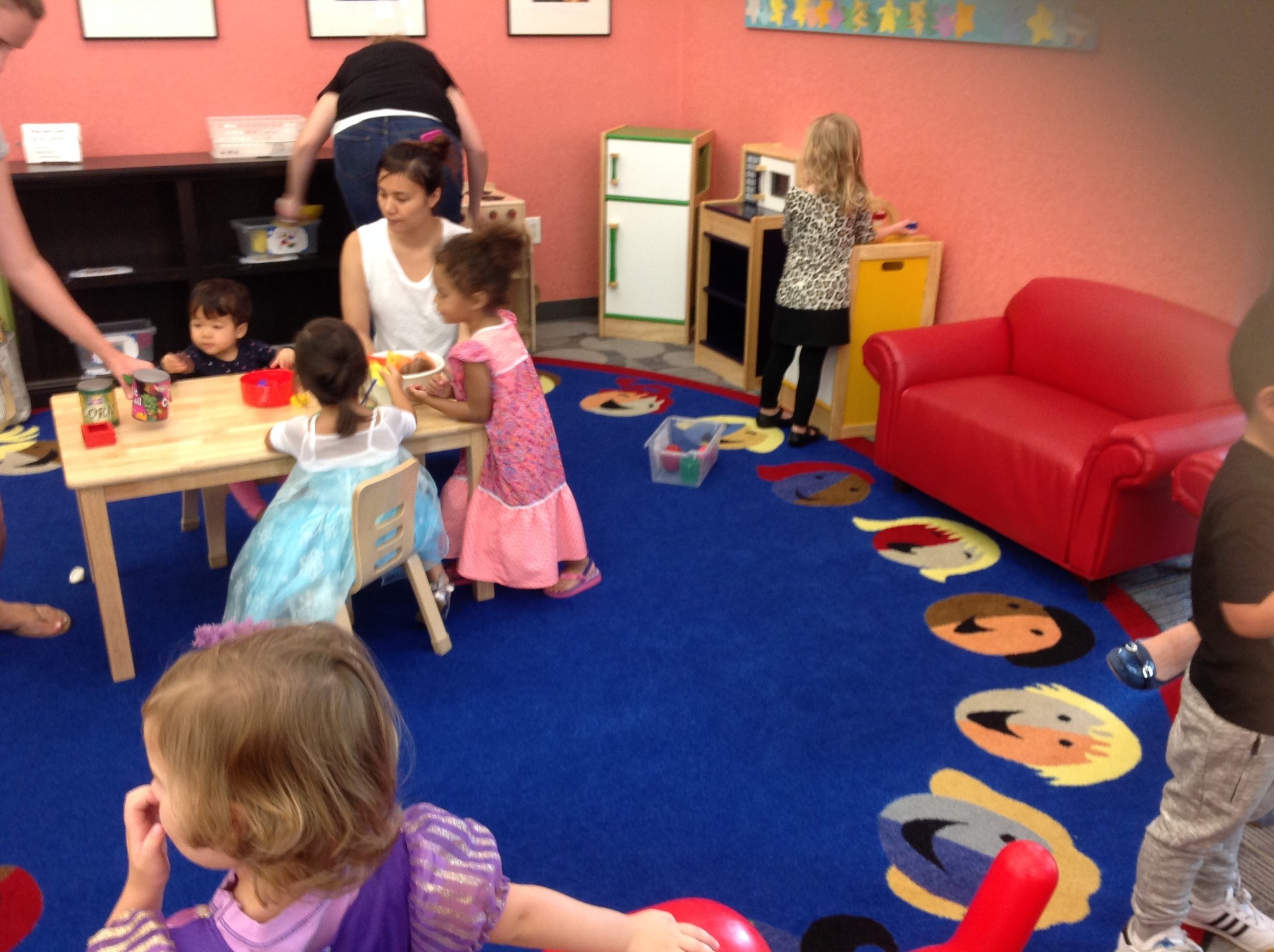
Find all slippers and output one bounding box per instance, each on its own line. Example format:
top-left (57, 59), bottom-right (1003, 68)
top-left (1, 603), bottom-right (71, 639)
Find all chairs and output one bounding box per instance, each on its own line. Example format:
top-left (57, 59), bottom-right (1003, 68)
top-left (329, 456), bottom-right (455, 656)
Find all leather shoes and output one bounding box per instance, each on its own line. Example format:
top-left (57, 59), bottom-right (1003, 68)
top-left (1109, 631), bottom-right (1187, 690)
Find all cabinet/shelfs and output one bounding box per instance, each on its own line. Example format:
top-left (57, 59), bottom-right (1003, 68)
top-left (598, 122), bottom-right (714, 346)
top-left (7, 151), bottom-right (362, 408)
top-left (460, 182), bottom-right (541, 353)
top-left (776, 237), bottom-right (942, 442)
top-left (696, 141), bottom-right (808, 388)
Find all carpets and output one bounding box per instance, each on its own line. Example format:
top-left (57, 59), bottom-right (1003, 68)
top-left (0, 355), bottom-right (1185, 949)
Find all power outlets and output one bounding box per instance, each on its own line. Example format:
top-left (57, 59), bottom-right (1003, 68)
top-left (524, 215), bottom-right (541, 246)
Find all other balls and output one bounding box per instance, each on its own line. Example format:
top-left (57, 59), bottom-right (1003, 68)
top-left (698, 445), bottom-right (716, 463)
top-left (679, 456), bottom-right (700, 482)
top-left (662, 445), bottom-right (682, 471)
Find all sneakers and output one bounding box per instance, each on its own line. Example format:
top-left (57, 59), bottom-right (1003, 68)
top-left (1180, 886), bottom-right (1274, 952)
top-left (1113, 913), bottom-right (1202, 952)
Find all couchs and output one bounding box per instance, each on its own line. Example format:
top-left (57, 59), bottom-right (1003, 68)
top-left (861, 276), bottom-right (1251, 578)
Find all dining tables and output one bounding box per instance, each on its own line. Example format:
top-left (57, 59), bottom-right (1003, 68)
top-left (50, 375), bottom-right (496, 681)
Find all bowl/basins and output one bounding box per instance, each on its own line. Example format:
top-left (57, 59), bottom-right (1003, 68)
top-left (240, 368), bottom-right (295, 407)
top-left (366, 349), bottom-right (446, 405)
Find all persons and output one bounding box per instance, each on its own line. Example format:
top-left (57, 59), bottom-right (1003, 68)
top-left (1114, 285), bottom-right (1274, 952)
top-left (1105, 614), bottom-right (1203, 691)
top-left (754, 111), bottom-right (919, 449)
top-left (84, 616), bottom-right (724, 952)
top-left (159, 277), bottom-right (297, 525)
top-left (273, 33), bottom-right (490, 231)
top-left (220, 317), bottom-right (455, 631)
top-left (404, 218), bottom-right (603, 599)
top-left (0, 0), bottom-right (156, 641)
top-left (338, 134), bottom-right (476, 359)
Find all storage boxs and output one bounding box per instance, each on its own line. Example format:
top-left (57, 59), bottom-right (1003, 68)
top-left (74, 323), bottom-right (156, 373)
top-left (643, 416), bottom-right (727, 491)
top-left (230, 216), bottom-right (321, 255)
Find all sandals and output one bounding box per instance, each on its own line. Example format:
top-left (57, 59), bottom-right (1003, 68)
top-left (416, 582), bottom-right (454, 623)
top-left (756, 406), bottom-right (793, 428)
top-left (543, 556), bottom-right (603, 598)
top-left (440, 561), bottom-right (474, 584)
top-left (789, 425), bottom-right (821, 448)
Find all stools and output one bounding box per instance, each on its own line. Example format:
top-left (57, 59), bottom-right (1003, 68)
top-left (183, 477), bottom-right (294, 568)
